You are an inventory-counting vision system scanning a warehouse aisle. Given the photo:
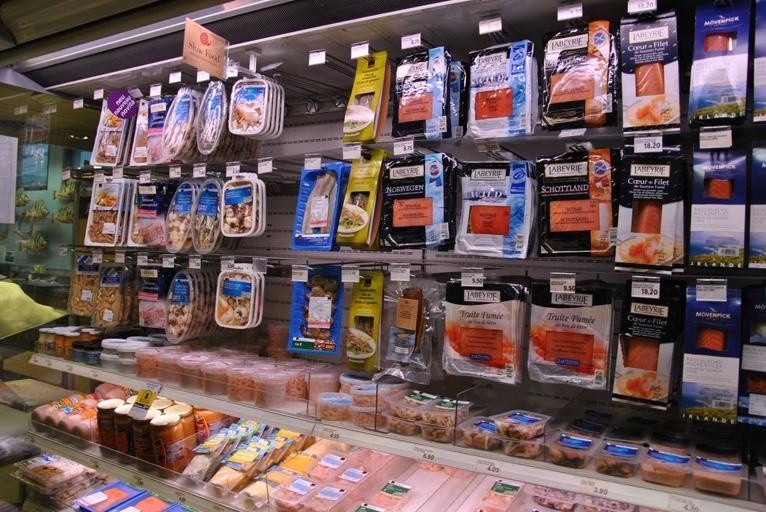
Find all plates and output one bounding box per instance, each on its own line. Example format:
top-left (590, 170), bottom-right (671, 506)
top-left (335, 204), bottom-right (371, 234)
top-left (346, 327), bottom-right (377, 365)
top-left (343, 105), bottom-right (374, 137)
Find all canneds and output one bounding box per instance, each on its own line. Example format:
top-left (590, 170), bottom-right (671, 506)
top-left (97, 393), bottom-right (240, 479)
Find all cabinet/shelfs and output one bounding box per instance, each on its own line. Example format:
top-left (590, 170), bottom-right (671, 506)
top-left (0, 10), bottom-right (766, 512)
